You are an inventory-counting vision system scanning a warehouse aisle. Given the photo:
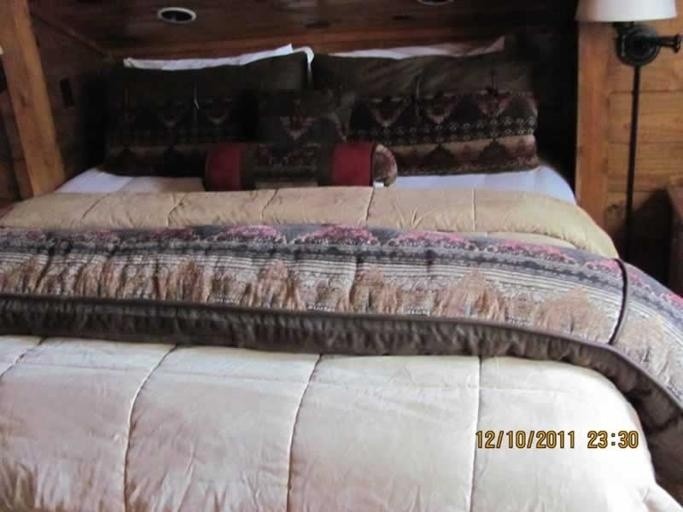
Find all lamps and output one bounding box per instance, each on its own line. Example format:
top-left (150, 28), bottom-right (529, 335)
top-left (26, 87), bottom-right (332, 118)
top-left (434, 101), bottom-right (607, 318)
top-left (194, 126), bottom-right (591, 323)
top-left (573, 0), bottom-right (682, 263)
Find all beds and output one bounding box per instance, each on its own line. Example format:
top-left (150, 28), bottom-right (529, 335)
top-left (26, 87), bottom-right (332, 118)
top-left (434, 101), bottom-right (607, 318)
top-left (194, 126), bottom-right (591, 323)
top-left (2, 152), bottom-right (680, 510)
